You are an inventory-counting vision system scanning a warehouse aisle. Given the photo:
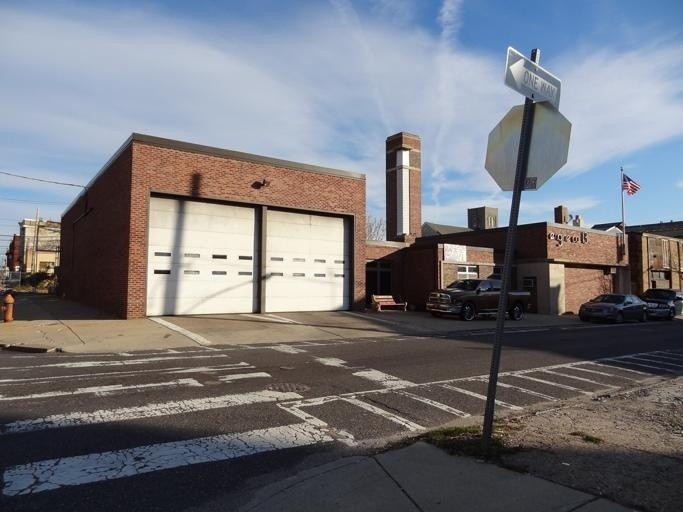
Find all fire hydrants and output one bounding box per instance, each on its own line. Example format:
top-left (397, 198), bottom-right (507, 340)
top-left (0, 292), bottom-right (15, 322)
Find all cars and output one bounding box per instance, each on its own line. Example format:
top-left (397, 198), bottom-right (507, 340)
top-left (577, 291), bottom-right (649, 326)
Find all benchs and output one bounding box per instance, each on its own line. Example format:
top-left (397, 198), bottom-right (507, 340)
top-left (371, 294), bottom-right (407, 312)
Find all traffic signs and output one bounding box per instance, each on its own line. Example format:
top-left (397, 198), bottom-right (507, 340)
top-left (499, 44), bottom-right (562, 115)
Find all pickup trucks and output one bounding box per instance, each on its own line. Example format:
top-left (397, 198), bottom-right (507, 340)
top-left (426, 277), bottom-right (533, 326)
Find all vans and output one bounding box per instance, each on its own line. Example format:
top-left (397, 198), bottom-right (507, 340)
top-left (640, 288), bottom-right (683, 320)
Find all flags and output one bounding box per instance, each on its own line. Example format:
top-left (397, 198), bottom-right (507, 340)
top-left (622, 173), bottom-right (640, 195)
